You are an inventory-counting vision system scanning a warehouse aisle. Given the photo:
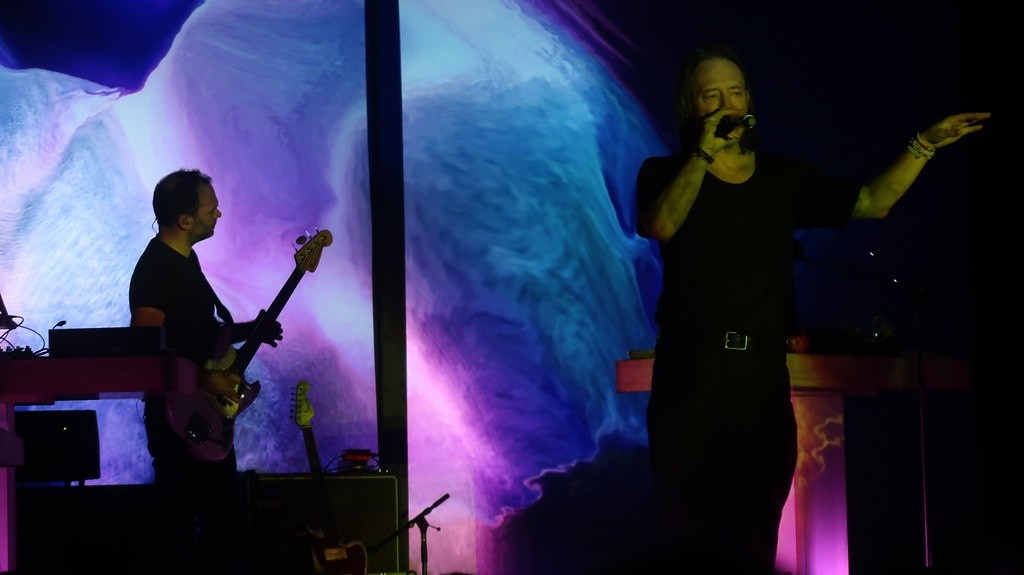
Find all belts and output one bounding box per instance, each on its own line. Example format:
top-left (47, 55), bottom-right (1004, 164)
top-left (659, 325), bottom-right (787, 357)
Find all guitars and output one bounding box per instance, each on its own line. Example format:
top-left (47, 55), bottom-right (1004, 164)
top-left (287, 379), bottom-right (371, 575)
top-left (162, 228), bottom-right (333, 461)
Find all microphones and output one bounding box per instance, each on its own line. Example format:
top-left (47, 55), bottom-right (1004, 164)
top-left (734, 115), bottom-right (756, 129)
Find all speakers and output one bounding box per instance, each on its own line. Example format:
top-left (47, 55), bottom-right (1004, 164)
top-left (250, 469), bottom-right (403, 575)
top-left (0, 0), bottom-right (205, 96)
top-left (13, 410), bottom-right (101, 483)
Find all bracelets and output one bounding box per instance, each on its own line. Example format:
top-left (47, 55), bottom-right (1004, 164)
top-left (691, 144), bottom-right (714, 164)
top-left (904, 130), bottom-right (936, 160)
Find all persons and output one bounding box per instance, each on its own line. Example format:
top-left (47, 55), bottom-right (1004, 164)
top-left (629, 42), bottom-right (991, 575)
top-left (130, 168), bottom-right (283, 483)
